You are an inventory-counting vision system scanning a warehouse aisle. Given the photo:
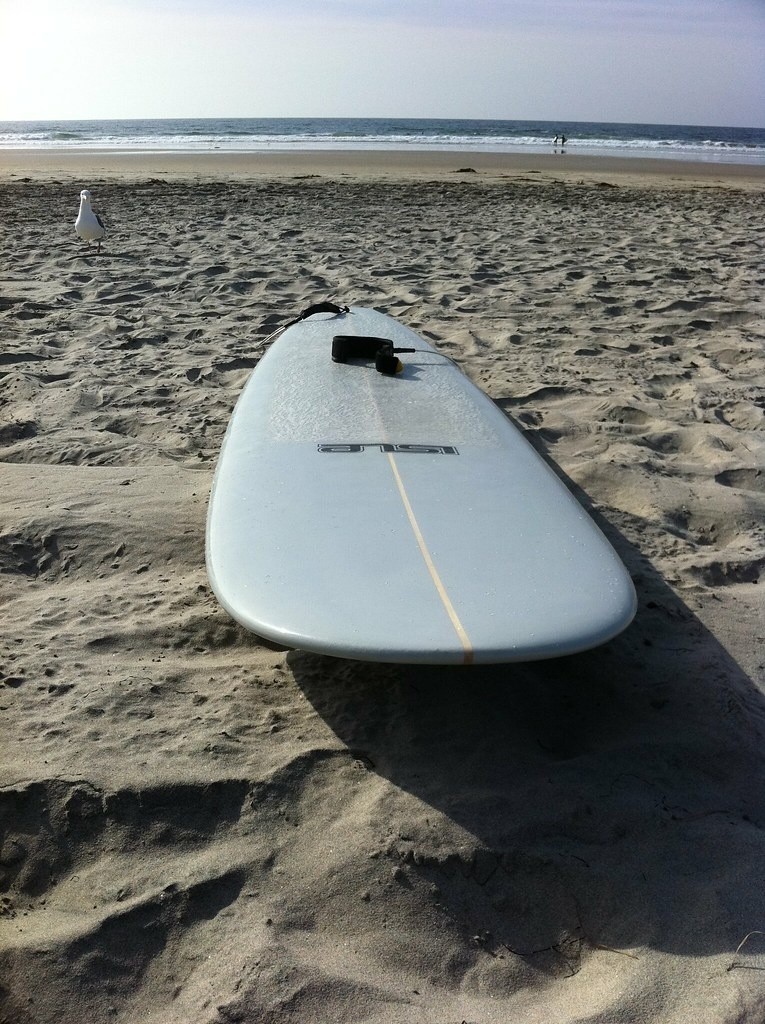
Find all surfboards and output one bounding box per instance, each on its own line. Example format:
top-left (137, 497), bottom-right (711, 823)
top-left (203, 306), bottom-right (639, 668)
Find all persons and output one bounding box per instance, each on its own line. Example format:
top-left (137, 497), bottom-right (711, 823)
top-left (554, 133), bottom-right (558, 143)
top-left (561, 135), bottom-right (565, 146)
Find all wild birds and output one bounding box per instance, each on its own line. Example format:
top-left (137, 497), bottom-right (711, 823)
top-left (74, 190), bottom-right (105, 254)
